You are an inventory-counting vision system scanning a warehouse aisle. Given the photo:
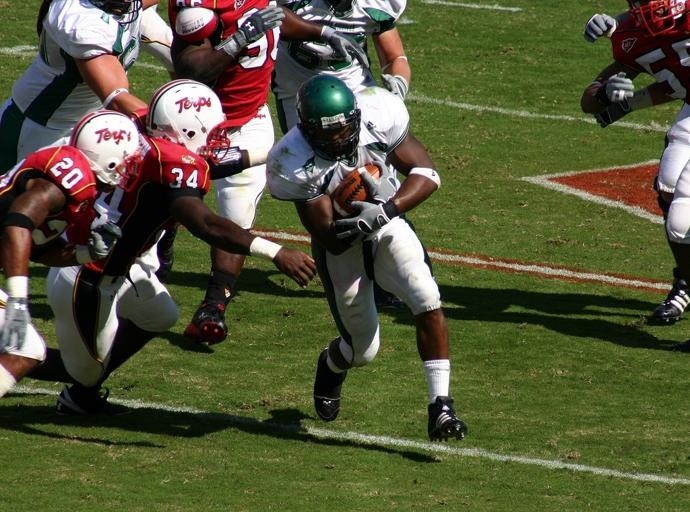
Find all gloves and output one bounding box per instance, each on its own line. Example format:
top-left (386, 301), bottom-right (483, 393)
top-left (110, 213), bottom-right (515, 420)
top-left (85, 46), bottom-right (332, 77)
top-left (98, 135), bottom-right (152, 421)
top-left (379, 72), bottom-right (409, 101)
top-left (0, 296), bottom-right (28, 349)
top-left (233, 5), bottom-right (286, 47)
top-left (584, 13), bottom-right (617, 43)
top-left (320, 25), bottom-right (371, 68)
top-left (332, 200), bottom-right (391, 246)
top-left (360, 160), bottom-right (398, 204)
top-left (83, 222), bottom-right (122, 263)
top-left (601, 71), bottom-right (634, 102)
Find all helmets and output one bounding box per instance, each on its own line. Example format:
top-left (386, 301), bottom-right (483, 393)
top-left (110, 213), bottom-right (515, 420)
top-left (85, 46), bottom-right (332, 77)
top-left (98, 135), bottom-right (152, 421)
top-left (294, 75), bottom-right (362, 161)
top-left (71, 108), bottom-right (144, 194)
top-left (627, 0), bottom-right (686, 37)
top-left (147, 78), bottom-right (231, 164)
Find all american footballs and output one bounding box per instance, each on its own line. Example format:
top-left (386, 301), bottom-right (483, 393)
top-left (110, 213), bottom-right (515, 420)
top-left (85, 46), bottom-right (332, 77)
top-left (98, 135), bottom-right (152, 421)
top-left (331, 163), bottom-right (382, 220)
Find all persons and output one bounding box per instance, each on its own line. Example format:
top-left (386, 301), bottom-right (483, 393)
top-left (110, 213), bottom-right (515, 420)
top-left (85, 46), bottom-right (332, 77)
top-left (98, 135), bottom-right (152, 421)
top-left (156, 0), bottom-right (369, 348)
top-left (1, 109), bottom-right (140, 399)
top-left (266, 75), bottom-right (467, 442)
top-left (580, 1), bottom-right (690, 325)
top-left (274, 0), bottom-right (411, 135)
top-left (47, 80), bottom-right (318, 418)
top-left (0, 1), bottom-right (175, 177)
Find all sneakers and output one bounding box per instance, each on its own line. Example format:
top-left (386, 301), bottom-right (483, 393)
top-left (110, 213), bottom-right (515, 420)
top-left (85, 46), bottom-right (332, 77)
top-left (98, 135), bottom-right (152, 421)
top-left (427, 395), bottom-right (467, 443)
top-left (313, 349), bottom-right (348, 422)
top-left (651, 279), bottom-right (690, 326)
top-left (180, 301), bottom-right (228, 346)
top-left (56, 385), bottom-right (128, 423)
